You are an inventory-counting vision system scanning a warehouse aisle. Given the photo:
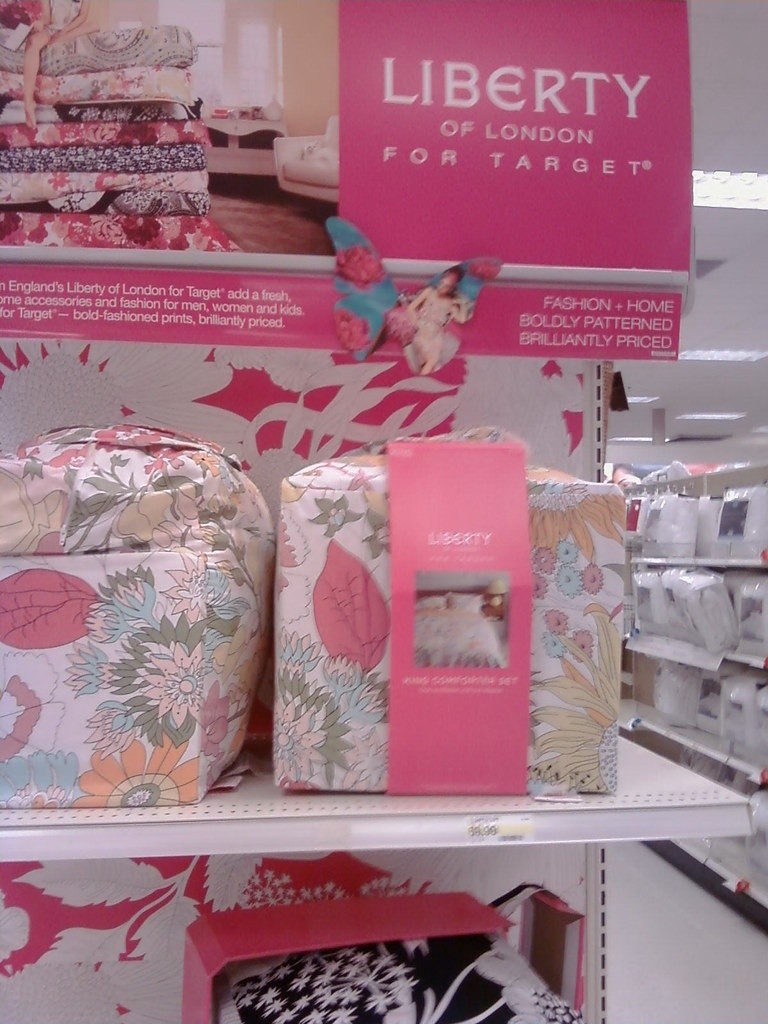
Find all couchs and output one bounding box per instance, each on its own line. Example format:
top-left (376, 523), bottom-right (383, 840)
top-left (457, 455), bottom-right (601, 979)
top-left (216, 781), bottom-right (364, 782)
top-left (273, 115), bottom-right (339, 202)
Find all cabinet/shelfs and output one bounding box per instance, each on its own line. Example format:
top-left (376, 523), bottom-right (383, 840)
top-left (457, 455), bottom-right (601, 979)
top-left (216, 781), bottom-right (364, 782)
top-left (0, 245), bottom-right (767, 1023)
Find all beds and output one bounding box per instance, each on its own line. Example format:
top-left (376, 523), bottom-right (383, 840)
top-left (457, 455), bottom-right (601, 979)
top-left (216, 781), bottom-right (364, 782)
top-left (413, 589), bottom-right (504, 669)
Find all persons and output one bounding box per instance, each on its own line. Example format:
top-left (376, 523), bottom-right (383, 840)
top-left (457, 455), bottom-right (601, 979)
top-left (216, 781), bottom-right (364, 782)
top-left (612, 463), bottom-right (661, 490)
top-left (23, 0), bottom-right (100, 130)
top-left (408, 266), bottom-right (466, 375)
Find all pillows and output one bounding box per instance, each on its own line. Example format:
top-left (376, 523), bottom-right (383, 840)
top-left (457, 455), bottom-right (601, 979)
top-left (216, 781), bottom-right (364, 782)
top-left (446, 592), bottom-right (483, 614)
top-left (414, 595), bottom-right (447, 610)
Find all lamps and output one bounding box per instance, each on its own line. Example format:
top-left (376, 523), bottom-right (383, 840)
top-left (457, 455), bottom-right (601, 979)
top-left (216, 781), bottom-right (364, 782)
top-left (486, 579), bottom-right (506, 607)
